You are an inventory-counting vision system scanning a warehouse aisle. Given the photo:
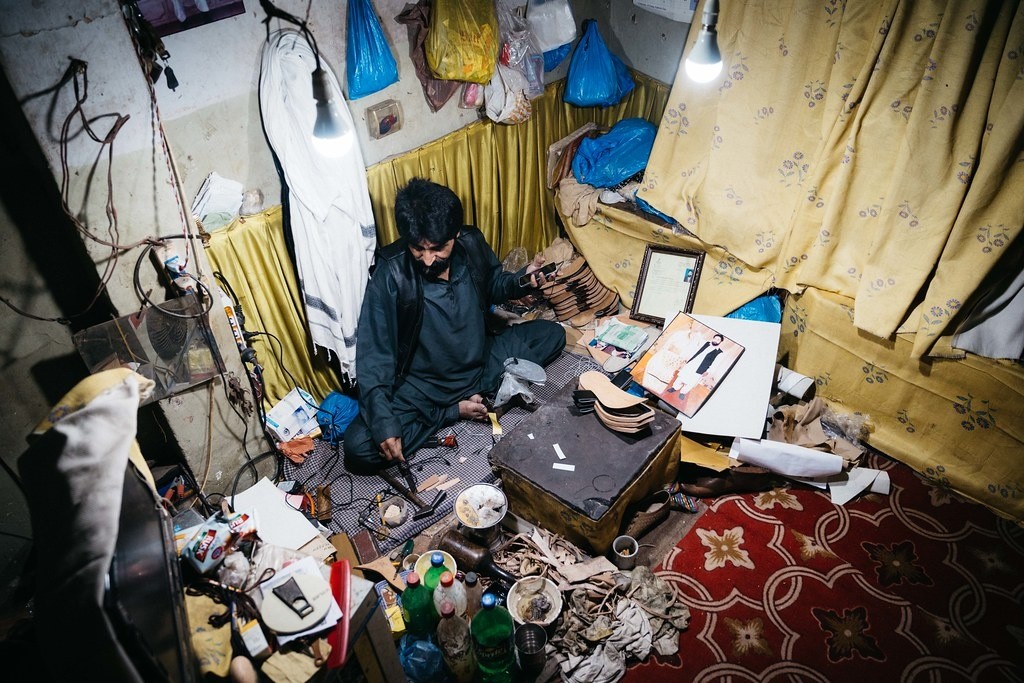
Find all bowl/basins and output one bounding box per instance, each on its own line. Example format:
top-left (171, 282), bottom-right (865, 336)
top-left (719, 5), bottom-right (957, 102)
top-left (506, 576), bottom-right (563, 627)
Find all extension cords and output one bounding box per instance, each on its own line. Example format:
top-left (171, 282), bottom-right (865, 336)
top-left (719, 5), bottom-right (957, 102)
top-left (151, 241), bottom-right (204, 308)
top-left (221, 292), bottom-right (248, 352)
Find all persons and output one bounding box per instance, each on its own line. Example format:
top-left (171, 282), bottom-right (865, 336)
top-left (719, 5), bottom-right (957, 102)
top-left (342, 176), bottom-right (566, 474)
top-left (683, 269), bottom-right (693, 283)
top-left (667, 335), bottom-right (724, 400)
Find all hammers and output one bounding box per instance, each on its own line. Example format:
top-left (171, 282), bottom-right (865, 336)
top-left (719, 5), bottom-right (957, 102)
top-left (378, 469), bottom-right (446, 521)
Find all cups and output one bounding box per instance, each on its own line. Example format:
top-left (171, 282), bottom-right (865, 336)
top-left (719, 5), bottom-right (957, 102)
top-left (513, 623), bottom-right (547, 674)
top-left (612, 535), bottom-right (639, 570)
top-left (414, 550), bottom-right (458, 589)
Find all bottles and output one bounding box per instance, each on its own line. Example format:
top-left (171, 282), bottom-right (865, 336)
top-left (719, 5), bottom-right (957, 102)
top-left (438, 530), bottom-right (515, 582)
top-left (402, 552), bottom-right (516, 683)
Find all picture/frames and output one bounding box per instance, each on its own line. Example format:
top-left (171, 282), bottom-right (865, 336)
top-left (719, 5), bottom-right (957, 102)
top-left (629, 242), bottom-right (707, 327)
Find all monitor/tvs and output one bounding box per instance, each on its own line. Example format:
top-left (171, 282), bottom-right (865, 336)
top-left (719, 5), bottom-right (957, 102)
top-left (46, 449), bottom-right (197, 683)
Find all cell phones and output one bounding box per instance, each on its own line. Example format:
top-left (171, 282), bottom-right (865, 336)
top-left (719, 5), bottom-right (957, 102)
top-left (518, 261), bottom-right (556, 287)
